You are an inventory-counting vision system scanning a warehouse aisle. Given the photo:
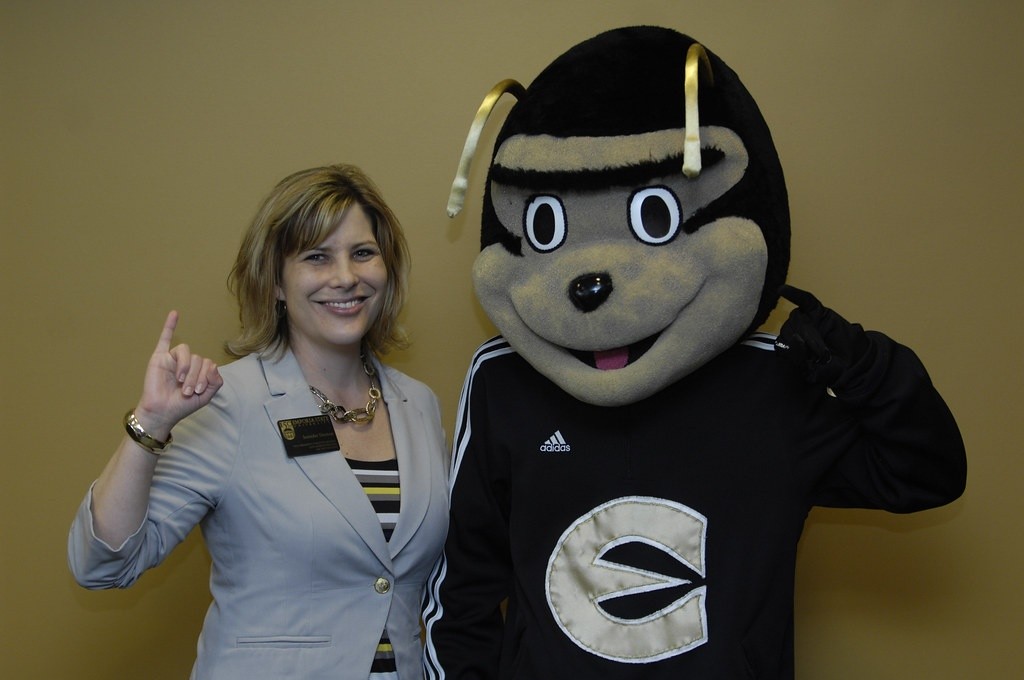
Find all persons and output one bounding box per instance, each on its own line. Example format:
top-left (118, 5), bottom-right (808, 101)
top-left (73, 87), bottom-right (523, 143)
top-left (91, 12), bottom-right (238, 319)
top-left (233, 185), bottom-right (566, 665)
top-left (66, 162), bottom-right (448, 680)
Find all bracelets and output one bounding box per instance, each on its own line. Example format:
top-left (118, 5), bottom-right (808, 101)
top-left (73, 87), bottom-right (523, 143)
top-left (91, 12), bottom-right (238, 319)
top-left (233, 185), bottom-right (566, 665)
top-left (121, 409), bottom-right (174, 454)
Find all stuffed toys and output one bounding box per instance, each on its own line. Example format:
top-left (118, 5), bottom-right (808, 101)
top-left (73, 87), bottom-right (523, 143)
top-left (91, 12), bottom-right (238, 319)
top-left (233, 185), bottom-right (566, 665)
top-left (418, 26), bottom-right (968, 680)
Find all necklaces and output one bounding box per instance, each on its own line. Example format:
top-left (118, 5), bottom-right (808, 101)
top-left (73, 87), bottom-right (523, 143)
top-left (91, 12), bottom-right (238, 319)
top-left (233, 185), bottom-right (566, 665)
top-left (305, 353), bottom-right (382, 423)
